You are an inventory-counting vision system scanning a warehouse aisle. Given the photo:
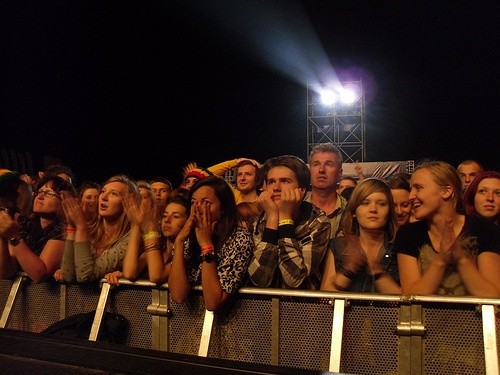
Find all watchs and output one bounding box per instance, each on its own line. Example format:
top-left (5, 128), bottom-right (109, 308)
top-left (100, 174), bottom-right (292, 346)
top-left (9, 234), bottom-right (24, 247)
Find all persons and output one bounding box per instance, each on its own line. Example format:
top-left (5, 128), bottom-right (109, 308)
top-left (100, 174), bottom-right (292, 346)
top-left (77, 182), bottom-right (102, 214)
top-left (168, 175), bottom-right (254, 311)
top-left (206, 158), bottom-right (264, 204)
top-left (463, 170), bottom-right (500, 219)
top-left (247, 155), bottom-right (331, 291)
top-left (175, 163), bottom-right (209, 190)
top-left (303, 142), bottom-right (348, 239)
top-left (338, 160), bottom-right (367, 200)
top-left (62, 175), bottom-right (142, 282)
top-left (235, 202), bottom-right (263, 232)
top-left (157, 187), bottom-right (191, 218)
top-left (0, 169), bottom-right (37, 279)
top-left (120, 192), bottom-right (191, 283)
top-left (0, 175), bottom-right (78, 283)
top-left (150, 178), bottom-right (173, 206)
top-left (52, 181), bottom-right (155, 285)
top-left (388, 175), bottom-right (420, 239)
top-left (320, 178), bottom-right (402, 295)
top-left (36, 164), bottom-right (77, 197)
top-left (455, 160), bottom-right (484, 214)
top-left (394, 162), bottom-right (500, 298)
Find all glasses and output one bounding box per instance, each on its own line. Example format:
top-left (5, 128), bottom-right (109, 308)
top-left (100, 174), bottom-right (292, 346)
top-left (35, 189), bottom-right (59, 199)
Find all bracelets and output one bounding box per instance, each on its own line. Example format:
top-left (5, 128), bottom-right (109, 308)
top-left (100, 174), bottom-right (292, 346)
top-left (65, 226), bottom-right (75, 232)
top-left (199, 254), bottom-right (215, 263)
top-left (201, 246), bottom-right (213, 254)
top-left (143, 231), bottom-right (161, 251)
top-left (279, 220), bottom-right (294, 226)
